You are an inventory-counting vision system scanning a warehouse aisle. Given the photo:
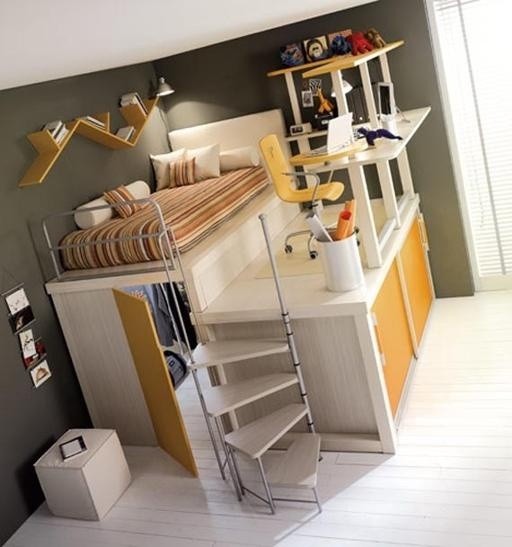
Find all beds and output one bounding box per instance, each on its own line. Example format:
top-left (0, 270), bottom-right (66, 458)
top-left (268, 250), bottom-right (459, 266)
top-left (41, 107), bottom-right (304, 314)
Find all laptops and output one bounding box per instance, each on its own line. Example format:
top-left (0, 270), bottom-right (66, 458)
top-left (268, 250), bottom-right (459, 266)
top-left (305, 111), bottom-right (354, 158)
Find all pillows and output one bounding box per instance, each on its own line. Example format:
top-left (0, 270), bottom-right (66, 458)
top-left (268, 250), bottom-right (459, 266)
top-left (74, 143), bottom-right (261, 230)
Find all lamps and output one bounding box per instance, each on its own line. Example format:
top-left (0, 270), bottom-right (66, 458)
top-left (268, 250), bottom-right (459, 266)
top-left (155, 77), bottom-right (175, 96)
top-left (330, 80), bottom-right (354, 98)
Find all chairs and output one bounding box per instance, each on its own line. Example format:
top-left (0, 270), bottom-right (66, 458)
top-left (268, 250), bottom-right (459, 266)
top-left (258, 134), bottom-right (345, 261)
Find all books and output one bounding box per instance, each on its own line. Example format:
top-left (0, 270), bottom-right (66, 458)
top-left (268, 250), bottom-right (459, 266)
top-left (58, 435), bottom-right (88, 462)
top-left (61, 439), bottom-right (81, 456)
top-left (40, 91), bottom-right (148, 146)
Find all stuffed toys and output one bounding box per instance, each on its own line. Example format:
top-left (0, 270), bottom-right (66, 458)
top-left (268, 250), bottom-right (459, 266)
top-left (315, 85), bottom-right (335, 113)
top-left (364, 28), bottom-right (386, 48)
top-left (344, 31), bottom-right (373, 56)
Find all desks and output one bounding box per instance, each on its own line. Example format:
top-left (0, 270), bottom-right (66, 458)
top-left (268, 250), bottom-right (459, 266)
top-left (33, 428), bottom-right (134, 521)
top-left (267, 40), bottom-right (431, 269)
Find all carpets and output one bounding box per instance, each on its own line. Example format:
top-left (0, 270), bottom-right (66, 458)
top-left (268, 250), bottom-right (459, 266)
top-left (256, 204), bottom-right (387, 277)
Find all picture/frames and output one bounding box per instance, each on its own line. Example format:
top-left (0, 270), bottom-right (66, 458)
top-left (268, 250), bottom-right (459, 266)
top-left (377, 81), bottom-right (412, 122)
